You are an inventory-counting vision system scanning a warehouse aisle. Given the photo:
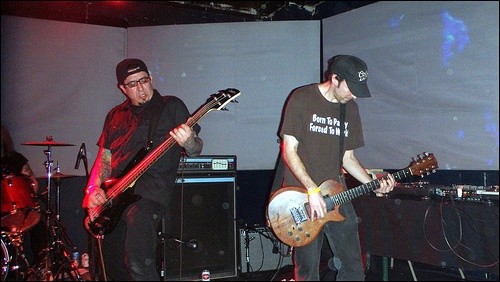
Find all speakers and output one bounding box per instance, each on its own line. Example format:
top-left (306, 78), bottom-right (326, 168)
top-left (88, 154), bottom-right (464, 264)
top-left (162, 177), bottom-right (236, 282)
top-left (240, 229), bottom-right (294, 274)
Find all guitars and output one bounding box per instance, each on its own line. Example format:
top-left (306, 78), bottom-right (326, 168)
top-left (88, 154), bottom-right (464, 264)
top-left (266, 152), bottom-right (438, 247)
top-left (84, 88), bottom-right (241, 239)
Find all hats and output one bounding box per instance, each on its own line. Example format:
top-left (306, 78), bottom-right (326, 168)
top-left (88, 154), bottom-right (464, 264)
top-left (116, 59), bottom-right (147, 84)
top-left (329, 54), bottom-right (372, 98)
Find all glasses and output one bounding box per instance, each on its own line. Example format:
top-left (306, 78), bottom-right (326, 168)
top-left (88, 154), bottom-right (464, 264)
top-left (123, 76), bottom-right (150, 88)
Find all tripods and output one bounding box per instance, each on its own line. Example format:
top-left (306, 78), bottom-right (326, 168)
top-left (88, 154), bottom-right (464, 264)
top-left (27, 146), bottom-right (88, 282)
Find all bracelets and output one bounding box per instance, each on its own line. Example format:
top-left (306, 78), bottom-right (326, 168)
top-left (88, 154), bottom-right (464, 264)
top-left (308, 187), bottom-right (320, 195)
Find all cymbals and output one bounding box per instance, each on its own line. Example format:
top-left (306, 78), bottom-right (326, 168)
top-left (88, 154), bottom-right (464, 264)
top-left (35, 173), bottom-right (79, 179)
top-left (20, 140), bottom-right (76, 147)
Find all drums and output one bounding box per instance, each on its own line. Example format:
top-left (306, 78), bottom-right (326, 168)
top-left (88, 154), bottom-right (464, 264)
top-left (0, 173), bottom-right (41, 233)
top-left (0, 231), bottom-right (16, 282)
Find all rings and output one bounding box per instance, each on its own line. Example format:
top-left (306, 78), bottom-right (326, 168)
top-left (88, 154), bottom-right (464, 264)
top-left (96, 193), bottom-right (101, 199)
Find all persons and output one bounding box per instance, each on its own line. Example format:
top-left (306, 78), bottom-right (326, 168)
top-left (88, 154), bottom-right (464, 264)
top-left (86, 58), bottom-right (203, 281)
top-left (0, 125), bottom-right (39, 270)
top-left (272, 55), bottom-right (394, 282)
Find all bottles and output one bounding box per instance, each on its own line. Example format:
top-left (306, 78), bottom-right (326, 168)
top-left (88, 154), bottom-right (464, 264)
top-left (71, 247), bottom-right (89, 267)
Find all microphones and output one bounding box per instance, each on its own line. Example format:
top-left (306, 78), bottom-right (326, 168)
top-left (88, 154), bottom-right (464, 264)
top-left (75, 143), bottom-right (84, 169)
top-left (190, 241), bottom-right (197, 249)
top-left (272, 240), bottom-right (279, 254)
